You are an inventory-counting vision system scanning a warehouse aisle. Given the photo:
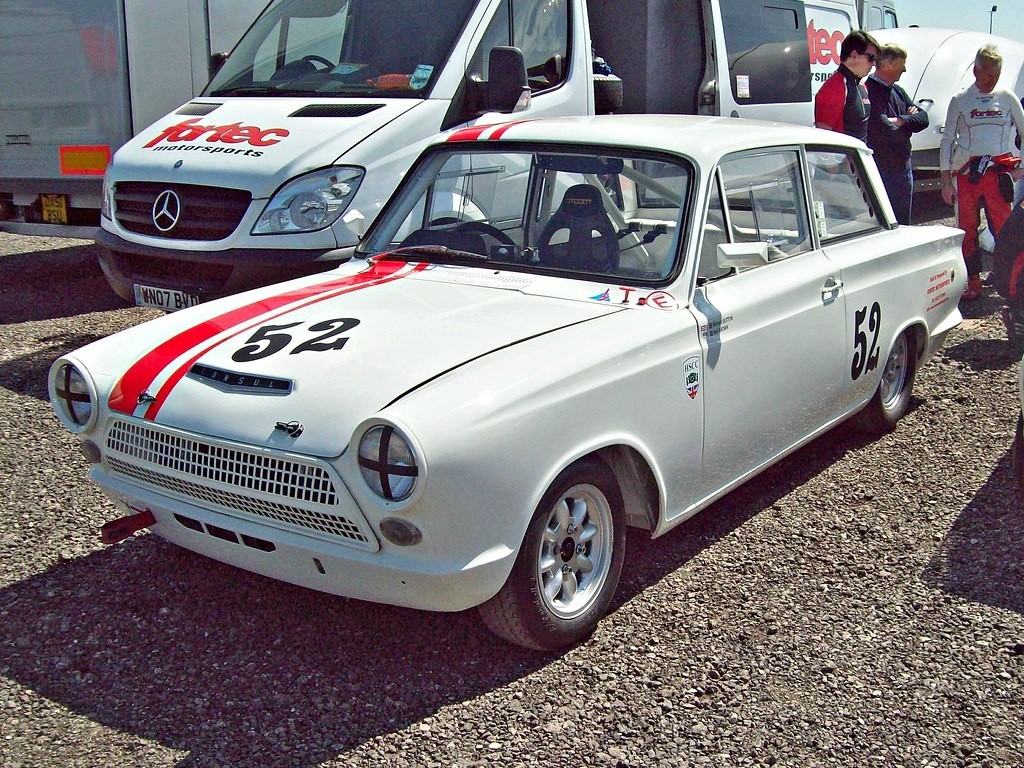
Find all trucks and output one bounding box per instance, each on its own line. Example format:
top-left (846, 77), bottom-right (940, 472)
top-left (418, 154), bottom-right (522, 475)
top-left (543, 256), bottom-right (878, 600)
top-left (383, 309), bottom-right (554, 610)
top-left (1, 0), bottom-right (347, 243)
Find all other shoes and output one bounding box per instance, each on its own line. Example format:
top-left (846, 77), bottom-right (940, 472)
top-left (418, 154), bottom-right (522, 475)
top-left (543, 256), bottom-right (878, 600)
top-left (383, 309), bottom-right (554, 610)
top-left (962, 275), bottom-right (982, 299)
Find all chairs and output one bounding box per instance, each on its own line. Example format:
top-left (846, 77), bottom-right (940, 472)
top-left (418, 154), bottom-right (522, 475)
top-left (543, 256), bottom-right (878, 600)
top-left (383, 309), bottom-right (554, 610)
top-left (535, 184), bottom-right (621, 274)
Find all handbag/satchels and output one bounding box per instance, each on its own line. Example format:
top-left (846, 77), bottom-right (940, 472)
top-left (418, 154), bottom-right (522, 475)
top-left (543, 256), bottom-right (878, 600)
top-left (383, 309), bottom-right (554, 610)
top-left (989, 151), bottom-right (1021, 172)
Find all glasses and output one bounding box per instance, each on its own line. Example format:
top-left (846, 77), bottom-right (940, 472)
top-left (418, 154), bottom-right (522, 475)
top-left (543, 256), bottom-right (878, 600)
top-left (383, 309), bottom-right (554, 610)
top-left (858, 51), bottom-right (877, 62)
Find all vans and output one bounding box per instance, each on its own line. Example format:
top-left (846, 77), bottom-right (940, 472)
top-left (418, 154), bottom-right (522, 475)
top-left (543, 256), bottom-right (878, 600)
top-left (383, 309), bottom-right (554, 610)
top-left (101, 2), bottom-right (865, 315)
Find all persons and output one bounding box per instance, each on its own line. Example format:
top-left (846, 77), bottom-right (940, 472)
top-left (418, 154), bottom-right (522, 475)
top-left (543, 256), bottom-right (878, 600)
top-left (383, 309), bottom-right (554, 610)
top-left (940, 43), bottom-right (1024, 301)
top-left (814, 31), bottom-right (882, 220)
top-left (865, 43), bottom-right (929, 226)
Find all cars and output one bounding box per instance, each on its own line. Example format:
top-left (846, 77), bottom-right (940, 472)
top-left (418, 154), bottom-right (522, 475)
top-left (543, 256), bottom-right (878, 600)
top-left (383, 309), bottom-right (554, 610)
top-left (44, 111), bottom-right (968, 653)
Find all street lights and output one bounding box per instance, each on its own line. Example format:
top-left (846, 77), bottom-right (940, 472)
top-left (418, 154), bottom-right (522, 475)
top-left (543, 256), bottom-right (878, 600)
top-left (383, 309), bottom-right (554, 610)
top-left (990, 5), bottom-right (1001, 32)
